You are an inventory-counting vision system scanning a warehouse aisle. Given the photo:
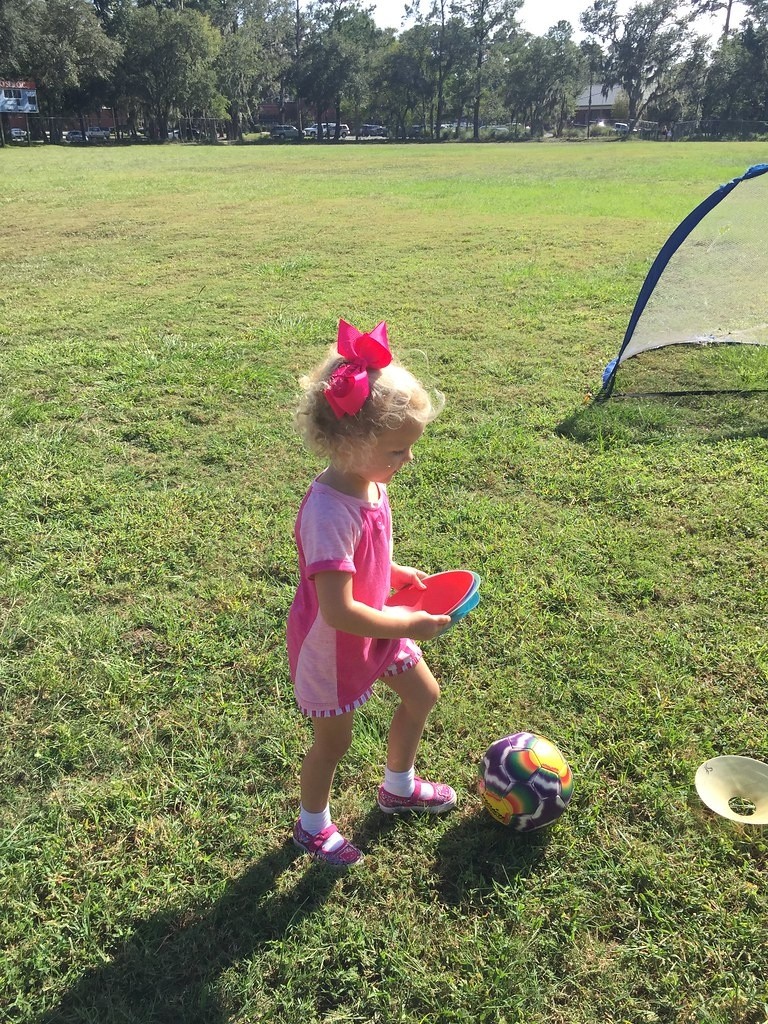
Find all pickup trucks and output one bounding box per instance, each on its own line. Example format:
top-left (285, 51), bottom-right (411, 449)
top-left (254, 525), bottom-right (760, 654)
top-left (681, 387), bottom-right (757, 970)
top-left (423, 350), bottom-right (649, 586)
top-left (103, 127), bottom-right (115, 139)
top-left (86, 127), bottom-right (104, 140)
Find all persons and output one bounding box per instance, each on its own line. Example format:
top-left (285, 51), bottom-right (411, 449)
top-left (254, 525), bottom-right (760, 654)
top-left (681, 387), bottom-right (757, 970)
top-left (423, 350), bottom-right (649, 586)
top-left (285, 319), bottom-right (456, 869)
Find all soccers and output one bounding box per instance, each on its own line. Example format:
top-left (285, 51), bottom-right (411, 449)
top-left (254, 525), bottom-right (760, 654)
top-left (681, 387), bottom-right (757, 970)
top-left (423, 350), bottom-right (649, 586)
top-left (476, 731), bottom-right (575, 832)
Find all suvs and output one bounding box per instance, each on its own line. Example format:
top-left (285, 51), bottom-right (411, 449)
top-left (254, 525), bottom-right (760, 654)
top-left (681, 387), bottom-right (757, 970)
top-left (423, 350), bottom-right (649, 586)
top-left (178, 130), bottom-right (204, 143)
top-left (322, 125), bottom-right (350, 138)
top-left (270, 125), bottom-right (304, 141)
top-left (360, 126), bottom-right (377, 137)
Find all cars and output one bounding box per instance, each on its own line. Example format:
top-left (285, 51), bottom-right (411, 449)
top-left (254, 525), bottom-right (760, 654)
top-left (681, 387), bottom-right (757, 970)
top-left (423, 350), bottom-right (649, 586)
top-left (11, 128), bottom-right (26, 139)
top-left (613, 122), bottom-right (638, 133)
top-left (138, 128), bottom-right (144, 134)
top-left (66, 131), bottom-right (86, 142)
top-left (434, 121), bottom-right (522, 131)
top-left (168, 130), bottom-right (179, 139)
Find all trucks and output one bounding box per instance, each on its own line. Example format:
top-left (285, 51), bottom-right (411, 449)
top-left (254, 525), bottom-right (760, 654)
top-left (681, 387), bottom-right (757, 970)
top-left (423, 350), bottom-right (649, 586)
top-left (304, 123), bottom-right (336, 137)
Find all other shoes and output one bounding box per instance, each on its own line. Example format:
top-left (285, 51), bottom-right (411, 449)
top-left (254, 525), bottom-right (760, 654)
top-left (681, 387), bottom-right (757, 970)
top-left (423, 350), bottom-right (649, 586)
top-left (292, 818), bottom-right (366, 869)
top-left (376, 778), bottom-right (457, 815)
